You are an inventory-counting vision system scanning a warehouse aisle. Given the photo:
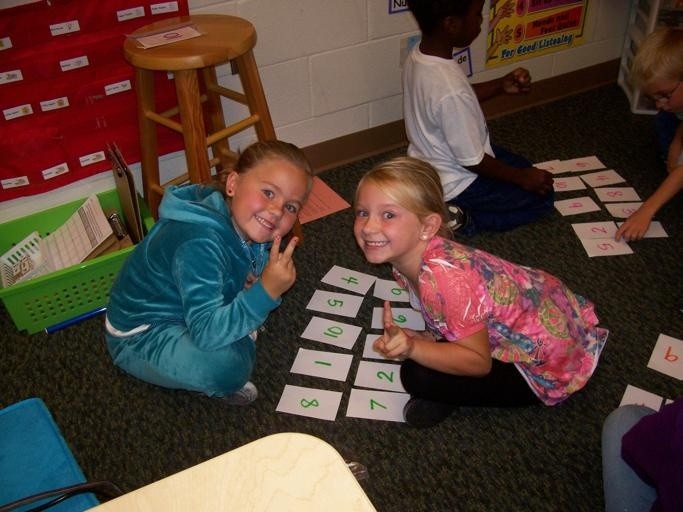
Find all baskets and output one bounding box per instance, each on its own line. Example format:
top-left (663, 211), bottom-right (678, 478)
top-left (0, 187), bottom-right (156, 336)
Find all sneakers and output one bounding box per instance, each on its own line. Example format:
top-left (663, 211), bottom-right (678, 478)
top-left (403, 395), bottom-right (455, 428)
top-left (222, 381), bottom-right (258, 405)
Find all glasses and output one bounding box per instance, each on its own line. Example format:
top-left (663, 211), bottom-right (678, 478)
top-left (645, 82), bottom-right (680, 104)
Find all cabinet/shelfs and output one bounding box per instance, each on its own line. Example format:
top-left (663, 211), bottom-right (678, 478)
top-left (616, 0), bottom-right (683, 116)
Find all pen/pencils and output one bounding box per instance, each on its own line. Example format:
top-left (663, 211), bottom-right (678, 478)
top-left (44, 306), bottom-right (108, 335)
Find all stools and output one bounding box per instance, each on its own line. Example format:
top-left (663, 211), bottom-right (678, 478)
top-left (122, 13), bottom-right (306, 247)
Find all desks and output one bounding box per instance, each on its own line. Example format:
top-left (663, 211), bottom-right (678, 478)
top-left (0, 432), bottom-right (378, 511)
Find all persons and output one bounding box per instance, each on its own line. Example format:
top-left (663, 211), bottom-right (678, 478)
top-left (350, 156), bottom-right (612, 429)
top-left (102, 139), bottom-right (316, 405)
top-left (613, 24), bottom-right (683, 244)
top-left (399, 1), bottom-right (557, 240)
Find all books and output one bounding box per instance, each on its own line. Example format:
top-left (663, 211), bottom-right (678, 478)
top-left (39, 191), bottom-right (116, 272)
